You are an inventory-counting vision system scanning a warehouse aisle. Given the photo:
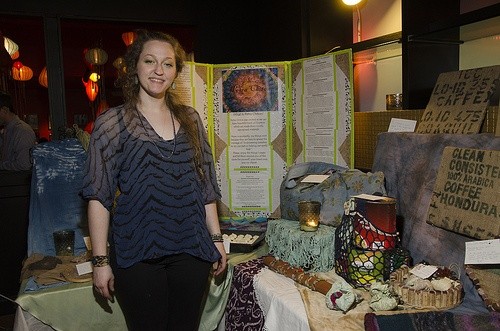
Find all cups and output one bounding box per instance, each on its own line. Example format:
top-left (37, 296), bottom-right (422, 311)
top-left (298, 200), bottom-right (321, 232)
top-left (386, 94), bottom-right (403, 110)
top-left (54, 229), bottom-right (76, 256)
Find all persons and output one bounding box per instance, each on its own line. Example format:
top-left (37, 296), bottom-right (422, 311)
top-left (80, 30), bottom-right (227, 331)
top-left (0, 92), bottom-right (38, 208)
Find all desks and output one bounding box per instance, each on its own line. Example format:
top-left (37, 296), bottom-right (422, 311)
top-left (13, 243), bottom-right (268, 331)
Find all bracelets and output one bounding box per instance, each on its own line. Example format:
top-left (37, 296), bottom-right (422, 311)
top-left (89, 255), bottom-right (112, 268)
top-left (209, 234), bottom-right (225, 242)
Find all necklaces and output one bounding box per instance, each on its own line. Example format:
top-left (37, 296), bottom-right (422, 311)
top-left (132, 101), bottom-right (176, 159)
